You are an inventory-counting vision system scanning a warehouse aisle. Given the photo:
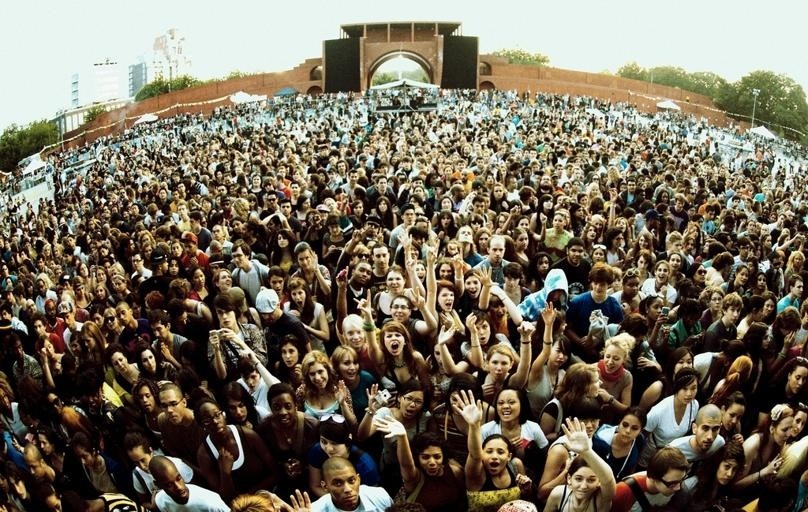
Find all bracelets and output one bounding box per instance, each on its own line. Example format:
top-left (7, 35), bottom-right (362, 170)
top-left (694, 338), bottom-right (745, 395)
top-left (779, 353), bottom-right (787, 359)
top-left (521, 341), bottom-right (531, 344)
top-left (364, 408), bottom-right (375, 415)
top-left (758, 471), bottom-right (760, 480)
top-left (543, 341), bottom-right (552, 345)
top-left (603, 395), bottom-right (614, 407)
top-left (471, 345), bottom-right (480, 348)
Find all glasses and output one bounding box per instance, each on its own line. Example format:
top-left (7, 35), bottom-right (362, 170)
top-left (403, 395), bottom-right (424, 407)
top-left (319, 413), bottom-right (345, 423)
top-left (593, 244), bottom-right (606, 250)
top-left (358, 253), bottom-right (369, 259)
top-left (623, 269), bottom-right (640, 278)
top-left (698, 270), bottom-right (707, 274)
top-left (132, 259), bottom-right (142, 262)
top-left (104, 316), bottom-right (116, 323)
top-left (202, 411), bottom-right (222, 425)
top-left (267, 198), bottom-right (276, 201)
top-left (796, 356), bottom-right (808, 363)
top-left (159, 398), bottom-right (183, 411)
top-left (470, 220), bottom-right (481, 225)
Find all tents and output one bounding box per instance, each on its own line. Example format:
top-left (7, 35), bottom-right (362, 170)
top-left (371, 78), bottom-right (436, 88)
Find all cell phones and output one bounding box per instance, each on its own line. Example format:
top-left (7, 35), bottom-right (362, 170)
top-left (663, 307), bottom-right (670, 318)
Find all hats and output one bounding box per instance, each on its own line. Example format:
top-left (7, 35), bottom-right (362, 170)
top-left (220, 195), bottom-right (231, 202)
top-left (179, 231), bottom-right (198, 243)
top-left (316, 205), bottom-right (331, 212)
top-left (256, 288), bottom-right (280, 314)
top-left (149, 248), bottom-right (167, 263)
top-left (58, 301), bottom-right (74, 316)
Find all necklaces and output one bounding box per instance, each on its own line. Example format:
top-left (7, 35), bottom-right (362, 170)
top-left (392, 359), bottom-right (408, 368)
top-left (274, 416), bottom-right (298, 446)
top-left (569, 494), bottom-right (590, 512)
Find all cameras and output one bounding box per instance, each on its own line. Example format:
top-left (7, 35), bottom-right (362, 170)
top-left (375, 388), bottom-right (392, 406)
top-left (217, 330), bottom-right (226, 341)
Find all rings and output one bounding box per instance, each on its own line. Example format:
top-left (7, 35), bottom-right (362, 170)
top-left (774, 463), bottom-right (776, 467)
